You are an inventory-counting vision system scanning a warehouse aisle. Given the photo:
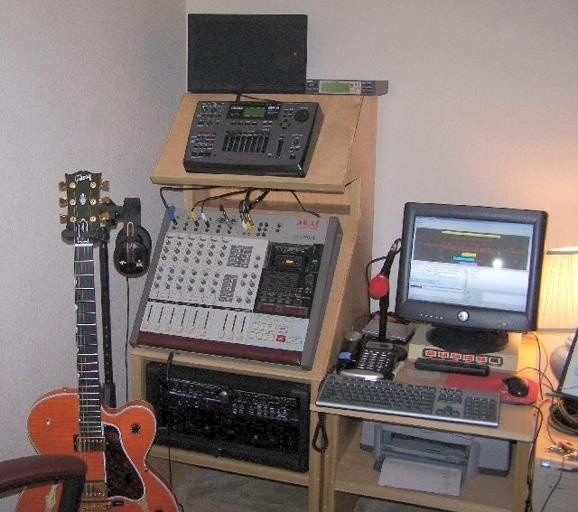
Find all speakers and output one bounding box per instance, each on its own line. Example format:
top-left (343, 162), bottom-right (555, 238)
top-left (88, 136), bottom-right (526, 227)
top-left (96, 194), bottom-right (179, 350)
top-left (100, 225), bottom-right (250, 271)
top-left (187, 13), bottom-right (309, 95)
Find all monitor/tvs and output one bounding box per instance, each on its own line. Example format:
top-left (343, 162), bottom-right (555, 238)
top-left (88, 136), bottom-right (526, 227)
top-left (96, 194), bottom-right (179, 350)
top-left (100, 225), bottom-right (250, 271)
top-left (395, 200), bottom-right (548, 355)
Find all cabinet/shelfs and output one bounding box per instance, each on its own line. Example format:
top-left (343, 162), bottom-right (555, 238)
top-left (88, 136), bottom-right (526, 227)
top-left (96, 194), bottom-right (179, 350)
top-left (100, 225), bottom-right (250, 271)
top-left (309, 398), bottom-right (541, 512)
top-left (127, 85), bottom-right (378, 512)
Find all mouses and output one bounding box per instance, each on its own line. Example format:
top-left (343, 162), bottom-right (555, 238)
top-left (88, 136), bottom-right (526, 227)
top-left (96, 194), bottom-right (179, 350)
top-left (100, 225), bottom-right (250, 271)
top-left (507, 375), bottom-right (528, 396)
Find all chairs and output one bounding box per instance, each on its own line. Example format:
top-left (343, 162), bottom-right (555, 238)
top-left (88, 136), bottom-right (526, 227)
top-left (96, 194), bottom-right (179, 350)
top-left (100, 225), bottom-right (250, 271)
top-left (0, 455), bottom-right (89, 512)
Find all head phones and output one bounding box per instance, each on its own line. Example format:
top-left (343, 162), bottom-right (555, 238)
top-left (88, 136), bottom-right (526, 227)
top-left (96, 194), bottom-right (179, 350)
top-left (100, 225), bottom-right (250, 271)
top-left (113, 198), bottom-right (151, 279)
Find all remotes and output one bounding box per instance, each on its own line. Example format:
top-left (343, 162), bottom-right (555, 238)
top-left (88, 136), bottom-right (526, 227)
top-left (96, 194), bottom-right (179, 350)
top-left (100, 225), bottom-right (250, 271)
top-left (415, 358), bottom-right (489, 376)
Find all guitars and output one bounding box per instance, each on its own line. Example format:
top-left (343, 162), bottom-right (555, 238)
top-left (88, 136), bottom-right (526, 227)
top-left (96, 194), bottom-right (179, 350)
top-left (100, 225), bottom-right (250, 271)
top-left (15, 171), bottom-right (183, 512)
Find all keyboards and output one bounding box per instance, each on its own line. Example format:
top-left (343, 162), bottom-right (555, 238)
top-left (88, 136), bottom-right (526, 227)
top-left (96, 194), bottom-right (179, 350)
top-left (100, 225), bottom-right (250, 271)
top-left (314, 374), bottom-right (500, 428)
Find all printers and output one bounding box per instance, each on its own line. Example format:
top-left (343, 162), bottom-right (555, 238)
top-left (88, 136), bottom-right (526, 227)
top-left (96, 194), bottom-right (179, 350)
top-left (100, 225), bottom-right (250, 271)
top-left (359, 419), bottom-right (513, 479)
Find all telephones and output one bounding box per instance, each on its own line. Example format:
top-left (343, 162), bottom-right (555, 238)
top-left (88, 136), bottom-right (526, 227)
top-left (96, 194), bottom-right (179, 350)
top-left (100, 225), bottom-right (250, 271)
top-left (337, 341), bottom-right (395, 379)
